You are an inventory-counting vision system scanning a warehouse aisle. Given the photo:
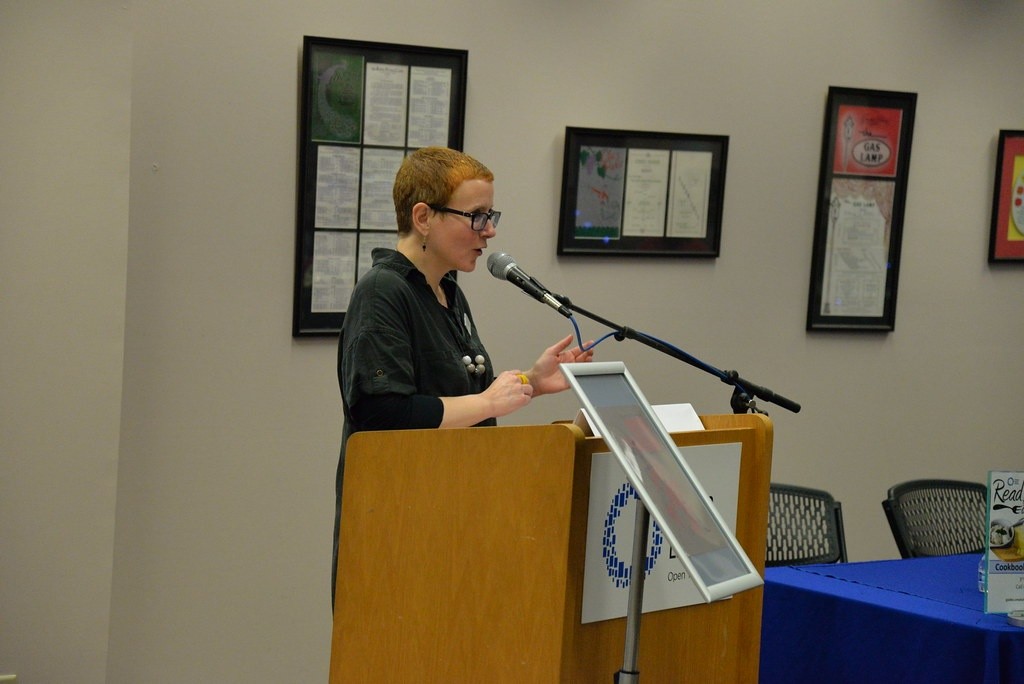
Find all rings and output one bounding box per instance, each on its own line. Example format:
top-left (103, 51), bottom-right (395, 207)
top-left (517, 374), bottom-right (529, 385)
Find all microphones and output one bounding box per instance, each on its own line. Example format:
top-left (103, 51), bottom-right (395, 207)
top-left (486, 251), bottom-right (572, 319)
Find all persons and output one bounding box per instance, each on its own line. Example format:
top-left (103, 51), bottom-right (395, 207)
top-left (331, 146), bottom-right (593, 614)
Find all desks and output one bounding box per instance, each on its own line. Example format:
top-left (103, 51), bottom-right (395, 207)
top-left (758, 553), bottom-right (1024, 684)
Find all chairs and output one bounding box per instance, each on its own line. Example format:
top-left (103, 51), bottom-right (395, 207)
top-left (764, 483), bottom-right (847, 567)
top-left (881, 478), bottom-right (987, 558)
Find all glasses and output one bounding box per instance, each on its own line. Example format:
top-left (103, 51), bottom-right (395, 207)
top-left (428, 203), bottom-right (501, 231)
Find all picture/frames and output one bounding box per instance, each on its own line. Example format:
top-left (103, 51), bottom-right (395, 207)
top-left (805, 85), bottom-right (918, 333)
top-left (987, 129), bottom-right (1024, 264)
top-left (291, 34), bottom-right (471, 342)
top-left (556, 125), bottom-right (732, 258)
